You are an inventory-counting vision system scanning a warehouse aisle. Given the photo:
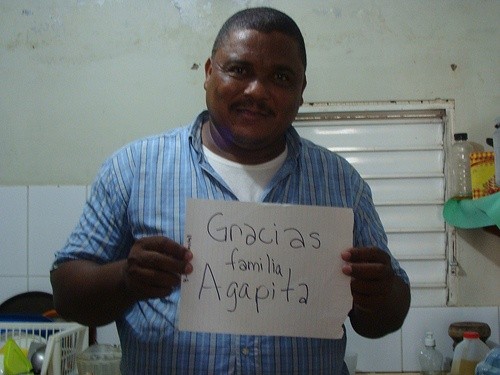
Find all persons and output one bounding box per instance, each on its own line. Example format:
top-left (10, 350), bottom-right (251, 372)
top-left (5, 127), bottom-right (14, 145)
top-left (48, 7), bottom-right (413, 374)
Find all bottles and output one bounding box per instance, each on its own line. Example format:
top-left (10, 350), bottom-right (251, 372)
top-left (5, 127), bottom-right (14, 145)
top-left (452, 330), bottom-right (493, 375)
top-left (448, 130), bottom-right (473, 198)
top-left (418, 329), bottom-right (445, 375)
